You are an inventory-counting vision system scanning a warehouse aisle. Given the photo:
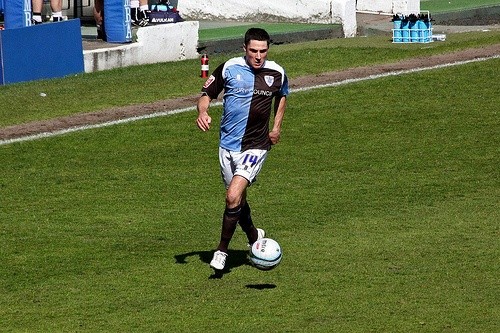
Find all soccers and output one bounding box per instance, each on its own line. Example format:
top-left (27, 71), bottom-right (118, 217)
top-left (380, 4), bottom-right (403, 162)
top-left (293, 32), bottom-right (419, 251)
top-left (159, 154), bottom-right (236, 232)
top-left (250, 238), bottom-right (280, 271)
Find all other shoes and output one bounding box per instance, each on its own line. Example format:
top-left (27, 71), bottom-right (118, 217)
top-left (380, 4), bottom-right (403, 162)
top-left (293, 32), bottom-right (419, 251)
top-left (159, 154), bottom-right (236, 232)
top-left (32, 15), bottom-right (43, 24)
top-left (49, 11), bottom-right (68, 22)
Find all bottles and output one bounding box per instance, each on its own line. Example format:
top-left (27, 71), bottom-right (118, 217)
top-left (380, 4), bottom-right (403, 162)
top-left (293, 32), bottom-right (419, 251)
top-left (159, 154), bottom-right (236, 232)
top-left (201, 54), bottom-right (209, 78)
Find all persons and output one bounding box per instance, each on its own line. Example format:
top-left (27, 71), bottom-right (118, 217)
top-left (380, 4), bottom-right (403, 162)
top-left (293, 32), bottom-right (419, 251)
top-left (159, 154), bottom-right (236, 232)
top-left (32, 0), bottom-right (67, 25)
top-left (196, 28), bottom-right (288, 270)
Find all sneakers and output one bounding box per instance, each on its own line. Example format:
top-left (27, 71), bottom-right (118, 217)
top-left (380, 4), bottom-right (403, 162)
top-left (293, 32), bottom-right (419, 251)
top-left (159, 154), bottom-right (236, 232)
top-left (246, 228), bottom-right (265, 264)
top-left (209, 250), bottom-right (228, 270)
